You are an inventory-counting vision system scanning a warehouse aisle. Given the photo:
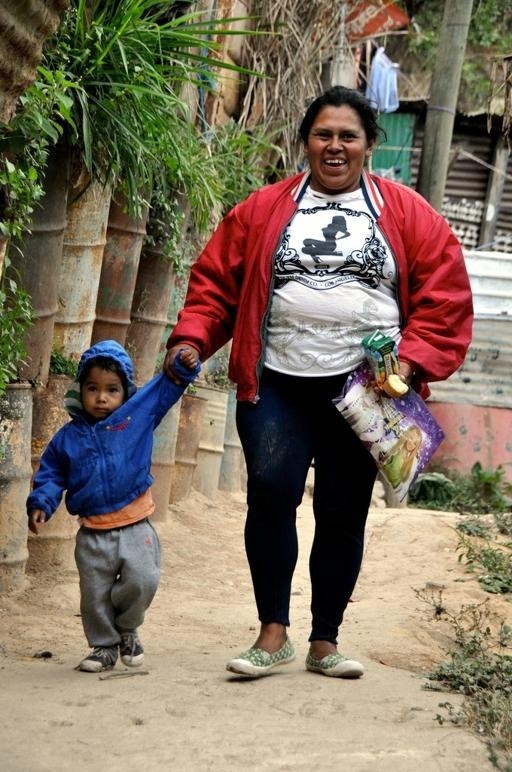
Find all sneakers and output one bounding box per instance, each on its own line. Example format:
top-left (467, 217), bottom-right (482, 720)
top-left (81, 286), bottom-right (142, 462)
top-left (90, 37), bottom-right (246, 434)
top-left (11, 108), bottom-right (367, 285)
top-left (226, 634), bottom-right (296, 675)
top-left (306, 650), bottom-right (364, 677)
top-left (114, 624), bottom-right (144, 666)
top-left (75, 643), bottom-right (118, 672)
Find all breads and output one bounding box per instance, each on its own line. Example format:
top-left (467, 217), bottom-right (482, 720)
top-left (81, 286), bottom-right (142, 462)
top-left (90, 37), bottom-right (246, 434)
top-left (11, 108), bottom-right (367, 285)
top-left (383, 374), bottom-right (409, 398)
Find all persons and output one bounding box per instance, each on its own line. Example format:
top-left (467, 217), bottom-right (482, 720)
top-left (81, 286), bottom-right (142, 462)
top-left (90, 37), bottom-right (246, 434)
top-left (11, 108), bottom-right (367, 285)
top-left (25, 339), bottom-right (201, 673)
top-left (163, 86), bottom-right (474, 679)
